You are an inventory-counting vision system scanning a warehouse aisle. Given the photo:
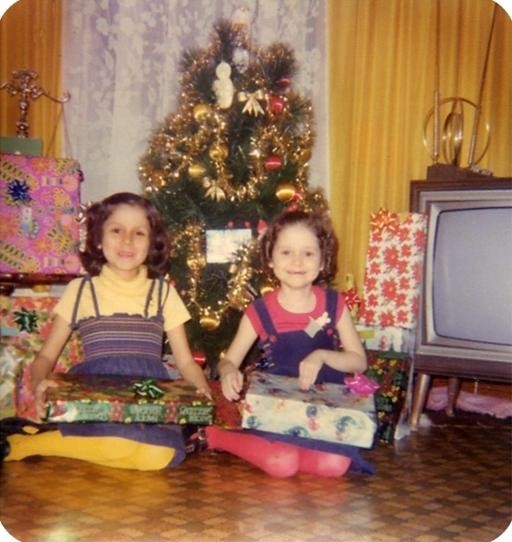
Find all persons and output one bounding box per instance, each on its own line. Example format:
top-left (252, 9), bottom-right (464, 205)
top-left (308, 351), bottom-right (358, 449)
top-left (0, 191), bottom-right (213, 471)
top-left (186, 210), bottom-right (368, 478)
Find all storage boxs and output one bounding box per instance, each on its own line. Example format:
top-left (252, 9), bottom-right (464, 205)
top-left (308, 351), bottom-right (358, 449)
top-left (36, 371), bottom-right (214, 426)
top-left (360, 210), bottom-right (428, 328)
top-left (236, 375), bottom-right (378, 447)
top-left (0, 156), bottom-right (81, 420)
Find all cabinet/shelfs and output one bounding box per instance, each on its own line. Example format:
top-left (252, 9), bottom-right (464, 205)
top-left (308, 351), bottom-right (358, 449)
top-left (409, 354), bottom-right (511, 438)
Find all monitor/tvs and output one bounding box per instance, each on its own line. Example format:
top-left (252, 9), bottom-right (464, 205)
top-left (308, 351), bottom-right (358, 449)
top-left (409, 176), bottom-right (512, 384)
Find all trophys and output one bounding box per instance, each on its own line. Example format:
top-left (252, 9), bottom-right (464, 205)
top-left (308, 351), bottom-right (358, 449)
top-left (0, 69), bottom-right (48, 155)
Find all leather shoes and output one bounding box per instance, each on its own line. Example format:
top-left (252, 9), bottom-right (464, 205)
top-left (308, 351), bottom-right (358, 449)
top-left (0, 416), bottom-right (44, 462)
top-left (182, 421), bottom-right (208, 455)
